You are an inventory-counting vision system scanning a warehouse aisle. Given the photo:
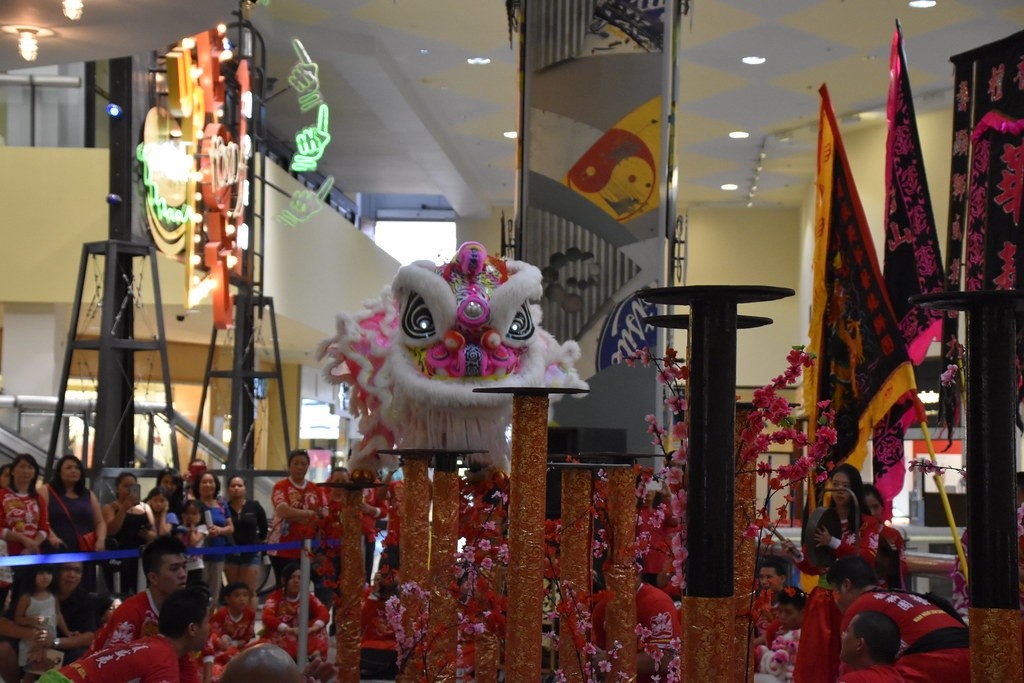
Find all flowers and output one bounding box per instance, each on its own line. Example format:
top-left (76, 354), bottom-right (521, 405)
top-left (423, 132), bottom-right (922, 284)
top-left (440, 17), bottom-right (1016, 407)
top-left (385, 336), bottom-right (1023, 683)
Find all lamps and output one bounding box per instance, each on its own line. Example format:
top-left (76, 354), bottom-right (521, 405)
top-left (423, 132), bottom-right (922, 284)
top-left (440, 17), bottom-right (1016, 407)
top-left (17, 28), bottom-right (38, 61)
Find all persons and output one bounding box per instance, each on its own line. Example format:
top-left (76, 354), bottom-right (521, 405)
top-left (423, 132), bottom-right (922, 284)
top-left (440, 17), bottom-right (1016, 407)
top-left (0, 452), bottom-right (158, 683)
top-left (37, 534), bottom-right (419, 682)
top-left (587, 553), bottom-right (684, 683)
top-left (752, 566), bottom-right (807, 649)
top-left (357, 546), bottom-right (498, 683)
top-left (1017, 470), bottom-right (1024, 509)
top-left (861, 482), bottom-right (909, 594)
top-left (266, 447), bottom-right (433, 638)
top-left (142, 458), bottom-right (269, 615)
top-left (829, 554), bottom-right (971, 683)
top-left (780, 464), bottom-right (879, 683)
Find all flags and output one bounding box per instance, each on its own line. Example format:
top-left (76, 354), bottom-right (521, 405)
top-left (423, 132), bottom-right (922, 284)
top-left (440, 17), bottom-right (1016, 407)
top-left (800, 102), bottom-right (914, 593)
top-left (871, 35), bottom-right (947, 526)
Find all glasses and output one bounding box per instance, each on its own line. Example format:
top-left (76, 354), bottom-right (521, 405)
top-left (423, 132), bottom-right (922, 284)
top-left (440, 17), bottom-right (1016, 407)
top-left (289, 449), bottom-right (307, 458)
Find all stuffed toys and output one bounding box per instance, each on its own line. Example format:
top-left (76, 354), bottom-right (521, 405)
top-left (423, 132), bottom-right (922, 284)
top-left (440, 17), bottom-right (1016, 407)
top-left (318, 240), bottom-right (587, 474)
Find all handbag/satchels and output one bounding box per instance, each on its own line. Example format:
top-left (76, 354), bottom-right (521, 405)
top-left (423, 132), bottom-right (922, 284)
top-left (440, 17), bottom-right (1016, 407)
top-left (79, 531), bottom-right (97, 551)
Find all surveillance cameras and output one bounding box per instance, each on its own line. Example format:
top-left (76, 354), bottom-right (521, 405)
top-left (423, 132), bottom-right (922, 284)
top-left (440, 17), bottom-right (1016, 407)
top-left (176, 315), bottom-right (184, 320)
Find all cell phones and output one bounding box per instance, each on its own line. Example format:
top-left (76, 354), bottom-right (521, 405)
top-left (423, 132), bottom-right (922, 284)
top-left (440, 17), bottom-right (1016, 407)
top-left (128, 484), bottom-right (140, 505)
